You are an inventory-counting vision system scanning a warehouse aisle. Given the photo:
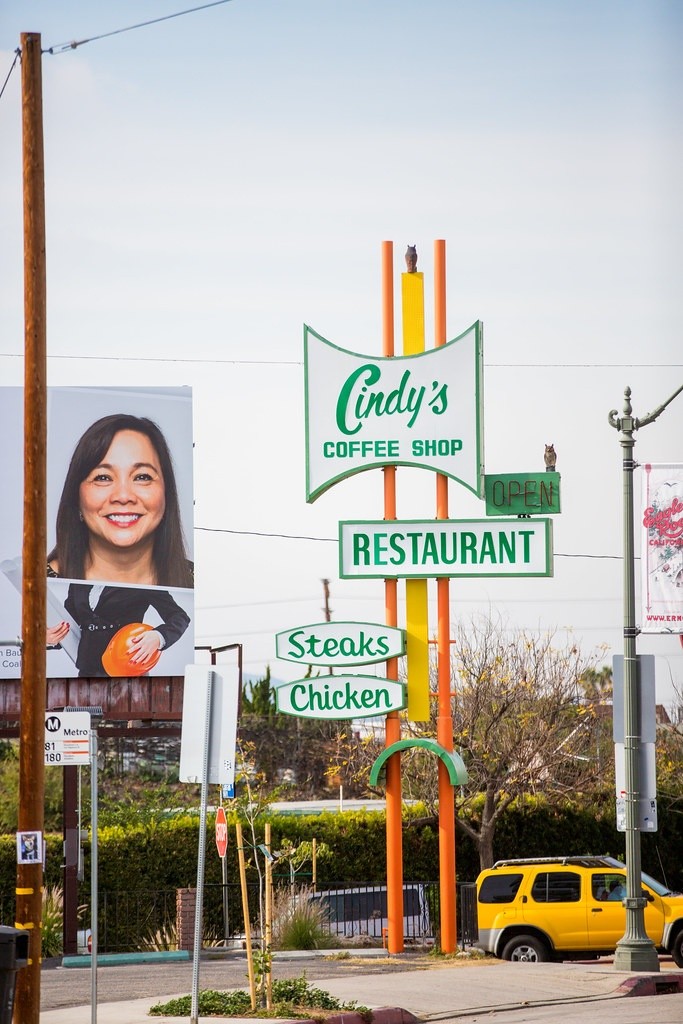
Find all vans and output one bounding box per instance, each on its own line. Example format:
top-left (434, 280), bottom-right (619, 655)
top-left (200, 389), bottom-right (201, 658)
top-left (223, 882), bottom-right (433, 948)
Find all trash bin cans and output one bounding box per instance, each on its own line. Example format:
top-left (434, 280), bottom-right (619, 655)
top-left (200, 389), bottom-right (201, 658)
top-left (0, 925), bottom-right (31, 1024)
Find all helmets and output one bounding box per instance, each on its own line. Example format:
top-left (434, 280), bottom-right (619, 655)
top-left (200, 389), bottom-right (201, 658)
top-left (102, 623), bottom-right (162, 676)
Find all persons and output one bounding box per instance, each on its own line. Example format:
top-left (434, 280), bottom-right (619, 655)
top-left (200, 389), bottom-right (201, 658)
top-left (47, 583), bottom-right (190, 676)
top-left (46, 414), bottom-right (194, 588)
top-left (601, 880), bottom-right (624, 900)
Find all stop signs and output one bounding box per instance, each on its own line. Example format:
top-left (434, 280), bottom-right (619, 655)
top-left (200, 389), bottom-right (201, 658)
top-left (215, 806), bottom-right (228, 858)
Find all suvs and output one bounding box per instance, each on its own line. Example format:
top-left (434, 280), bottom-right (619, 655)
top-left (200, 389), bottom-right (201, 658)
top-left (458, 854), bottom-right (683, 969)
